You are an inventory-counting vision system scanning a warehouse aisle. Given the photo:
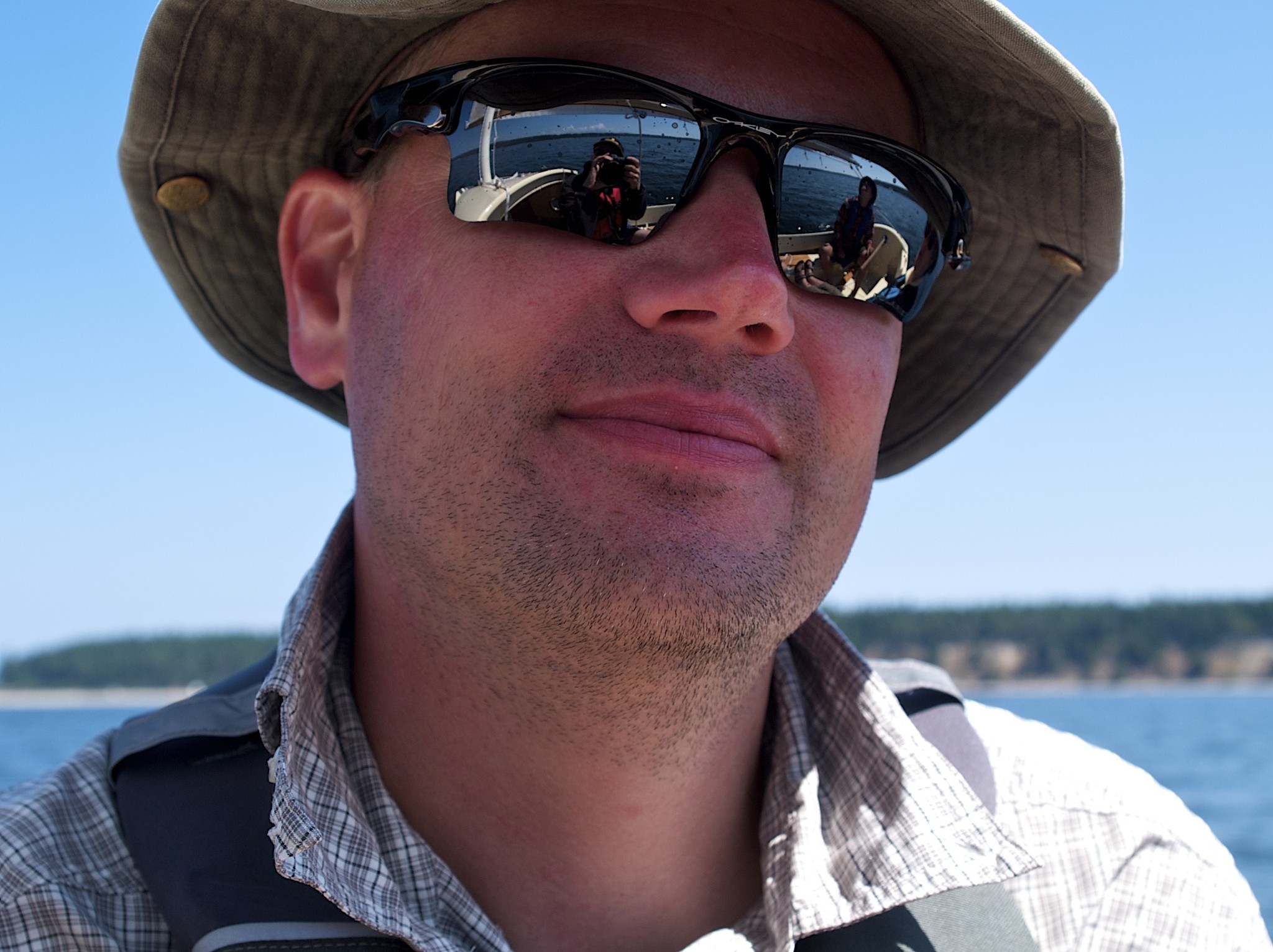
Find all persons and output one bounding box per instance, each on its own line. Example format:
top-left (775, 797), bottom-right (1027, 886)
top-left (795, 175), bottom-right (936, 318)
top-left (1, 0), bottom-right (1273, 952)
top-left (546, 133), bottom-right (655, 247)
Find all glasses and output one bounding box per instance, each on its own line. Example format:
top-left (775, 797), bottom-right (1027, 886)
top-left (334, 55), bottom-right (972, 325)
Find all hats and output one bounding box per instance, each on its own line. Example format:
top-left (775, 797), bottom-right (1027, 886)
top-left (859, 176), bottom-right (877, 206)
top-left (115, 0), bottom-right (1123, 482)
top-left (595, 137), bottom-right (623, 157)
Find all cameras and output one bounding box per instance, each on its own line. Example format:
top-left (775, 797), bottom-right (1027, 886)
top-left (603, 155), bottom-right (636, 180)
